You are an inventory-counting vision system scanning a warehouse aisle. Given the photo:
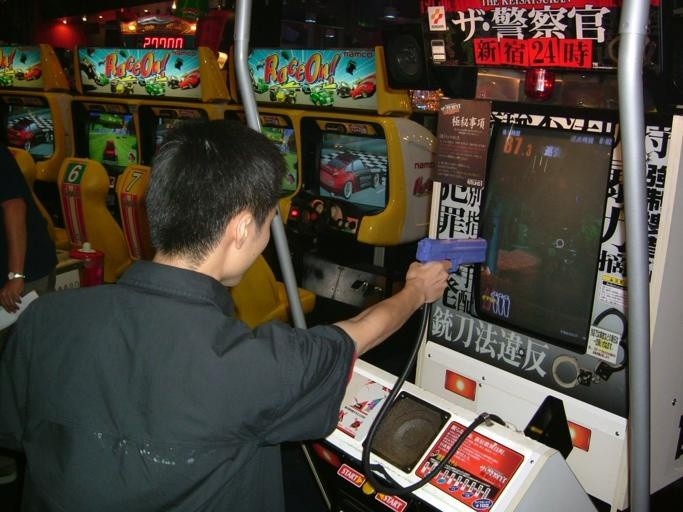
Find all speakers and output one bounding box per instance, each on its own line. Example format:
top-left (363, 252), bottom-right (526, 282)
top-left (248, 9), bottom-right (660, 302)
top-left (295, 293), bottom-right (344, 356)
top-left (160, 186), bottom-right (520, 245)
top-left (382, 22), bottom-right (431, 92)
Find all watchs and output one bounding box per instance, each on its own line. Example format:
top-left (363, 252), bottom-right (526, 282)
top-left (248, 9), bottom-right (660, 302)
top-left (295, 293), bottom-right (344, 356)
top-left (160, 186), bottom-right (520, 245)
top-left (6, 271), bottom-right (26, 280)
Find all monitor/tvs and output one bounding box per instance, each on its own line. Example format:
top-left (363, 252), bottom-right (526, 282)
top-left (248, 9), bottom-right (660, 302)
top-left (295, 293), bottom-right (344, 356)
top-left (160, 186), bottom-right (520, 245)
top-left (316, 132), bottom-right (389, 209)
top-left (0, 104), bottom-right (54, 157)
top-left (260, 125), bottom-right (298, 192)
top-left (469, 121), bottom-right (614, 354)
top-left (83, 108), bottom-right (139, 176)
top-left (150, 117), bottom-right (187, 167)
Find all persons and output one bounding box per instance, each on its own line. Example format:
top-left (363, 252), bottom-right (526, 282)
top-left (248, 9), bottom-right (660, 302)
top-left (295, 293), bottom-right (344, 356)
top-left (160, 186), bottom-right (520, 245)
top-left (0, 140), bottom-right (57, 484)
top-left (0, 119), bottom-right (452, 511)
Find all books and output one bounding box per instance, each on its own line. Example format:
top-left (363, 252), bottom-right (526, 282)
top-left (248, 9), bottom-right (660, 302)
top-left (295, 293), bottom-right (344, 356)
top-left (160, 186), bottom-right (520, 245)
top-left (0, 290), bottom-right (40, 351)
